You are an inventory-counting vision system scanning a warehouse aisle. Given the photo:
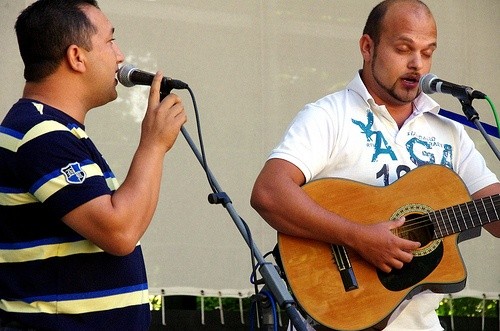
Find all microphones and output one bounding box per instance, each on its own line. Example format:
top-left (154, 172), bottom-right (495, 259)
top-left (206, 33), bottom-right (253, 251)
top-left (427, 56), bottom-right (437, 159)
top-left (419, 73), bottom-right (486, 100)
top-left (118, 65), bottom-right (188, 90)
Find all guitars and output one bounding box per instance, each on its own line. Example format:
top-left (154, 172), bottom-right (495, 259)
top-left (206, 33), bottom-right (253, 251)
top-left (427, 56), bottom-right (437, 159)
top-left (278, 164), bottom-right (500, 331)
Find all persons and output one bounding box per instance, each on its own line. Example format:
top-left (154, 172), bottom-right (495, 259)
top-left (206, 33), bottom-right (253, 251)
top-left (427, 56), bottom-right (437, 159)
top-left (250, 0), bottom-right (500, 331)
top-left (1, 0), bottom-right (187, 331)
